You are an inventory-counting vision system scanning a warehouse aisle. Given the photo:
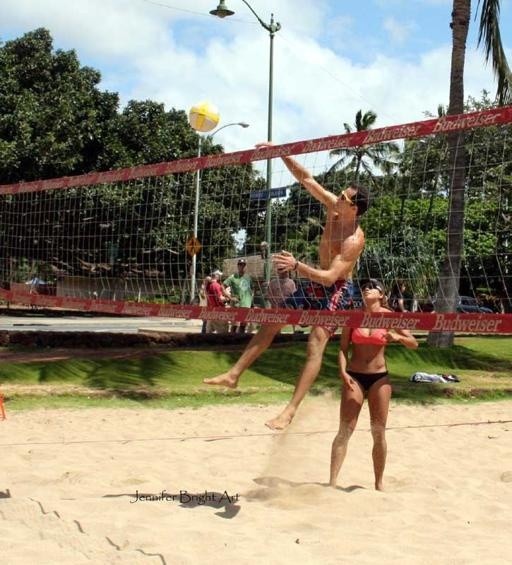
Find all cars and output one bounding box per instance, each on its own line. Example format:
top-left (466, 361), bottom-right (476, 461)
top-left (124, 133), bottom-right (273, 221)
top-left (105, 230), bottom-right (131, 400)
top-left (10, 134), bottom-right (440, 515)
top-left (422, 296), bottom-right (493, 313)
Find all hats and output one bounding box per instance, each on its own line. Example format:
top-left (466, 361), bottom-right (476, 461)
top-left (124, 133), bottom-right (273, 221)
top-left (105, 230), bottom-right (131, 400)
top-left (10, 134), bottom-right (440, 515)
top-left (357, 278), bottom-right (386, 296)
top-left (209, 270), bottom-right (223, 276)
top-left (237, 259), bottom-right (247, 265)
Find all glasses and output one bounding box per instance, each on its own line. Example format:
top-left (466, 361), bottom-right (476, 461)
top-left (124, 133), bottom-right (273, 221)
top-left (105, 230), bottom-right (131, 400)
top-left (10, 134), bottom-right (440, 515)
top-left (340, 190), bottom-right (352, 204)
top-left (361, 283), bottom-right (377, 292)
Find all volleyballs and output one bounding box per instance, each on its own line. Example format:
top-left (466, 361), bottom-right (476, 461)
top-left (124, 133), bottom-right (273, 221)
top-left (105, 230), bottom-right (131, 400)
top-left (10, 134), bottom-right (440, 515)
top-left (190, 101), bottom-right (220, 132)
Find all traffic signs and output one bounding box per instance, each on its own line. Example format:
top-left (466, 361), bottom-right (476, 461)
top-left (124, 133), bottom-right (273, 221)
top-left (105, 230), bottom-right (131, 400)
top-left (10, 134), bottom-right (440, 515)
top-left (247, 185), bottom-right (285, 199)
top-left (249, 199), bottom-right (267, 211)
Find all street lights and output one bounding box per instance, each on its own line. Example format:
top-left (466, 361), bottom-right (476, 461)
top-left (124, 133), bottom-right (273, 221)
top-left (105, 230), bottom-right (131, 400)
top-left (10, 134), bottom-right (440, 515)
top-left (189, 120), bottom-right (250, 304)
top-left (209, 0), bottom-right (281, 309)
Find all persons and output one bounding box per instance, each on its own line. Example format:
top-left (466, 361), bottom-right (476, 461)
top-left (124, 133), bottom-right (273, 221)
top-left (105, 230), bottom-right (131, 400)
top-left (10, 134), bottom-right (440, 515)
top-left (478, 294), bottom-right (504, 314)
top-left (385, 279), bottom-right (418, 313)
top-left (267, 268), bottom-right (297, 308)
top-left (198, 259), bottom-right (263, 336)
top-left (327, 276), bottom-right (419, 493)
top-left (197, 134), bottom-right (369, 435)
